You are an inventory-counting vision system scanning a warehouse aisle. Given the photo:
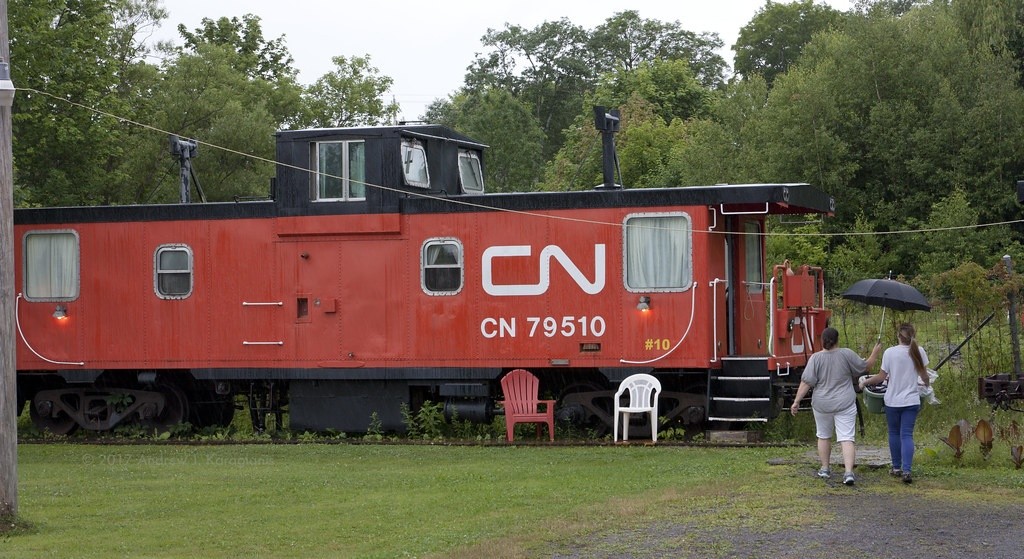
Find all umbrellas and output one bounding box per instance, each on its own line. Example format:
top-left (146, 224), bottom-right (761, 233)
top-left (841, 270), bottom-right (931, 345)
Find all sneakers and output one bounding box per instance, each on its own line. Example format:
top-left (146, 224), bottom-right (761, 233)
top-left (840, 472), bottom-right (855, 484)
top-left (816, 468), bottom-right (832, 478)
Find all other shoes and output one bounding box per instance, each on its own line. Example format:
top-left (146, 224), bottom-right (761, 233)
top-left (901, 473), bottom-right (914, 483)
top-left (888, 468), bottom-right (904, 477)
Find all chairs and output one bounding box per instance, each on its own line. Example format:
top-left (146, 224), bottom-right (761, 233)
top-left (614, 373), bottom-right (661, 442)
top-left (497, 369), bottom-right (556, 442)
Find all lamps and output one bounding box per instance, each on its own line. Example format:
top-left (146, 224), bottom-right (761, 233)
top-left (636, 296), bottom-right (650, 311)
top-left (52, 304), bottom-right (66, 320)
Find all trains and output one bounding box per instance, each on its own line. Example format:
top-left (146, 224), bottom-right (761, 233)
top-left (11, 103), bottom-right (838, 437)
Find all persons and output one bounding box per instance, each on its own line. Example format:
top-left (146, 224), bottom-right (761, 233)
top-left (791, 327), bottom-right (882, 485)
top-left (859, 323), bottom-right (929, 483)
